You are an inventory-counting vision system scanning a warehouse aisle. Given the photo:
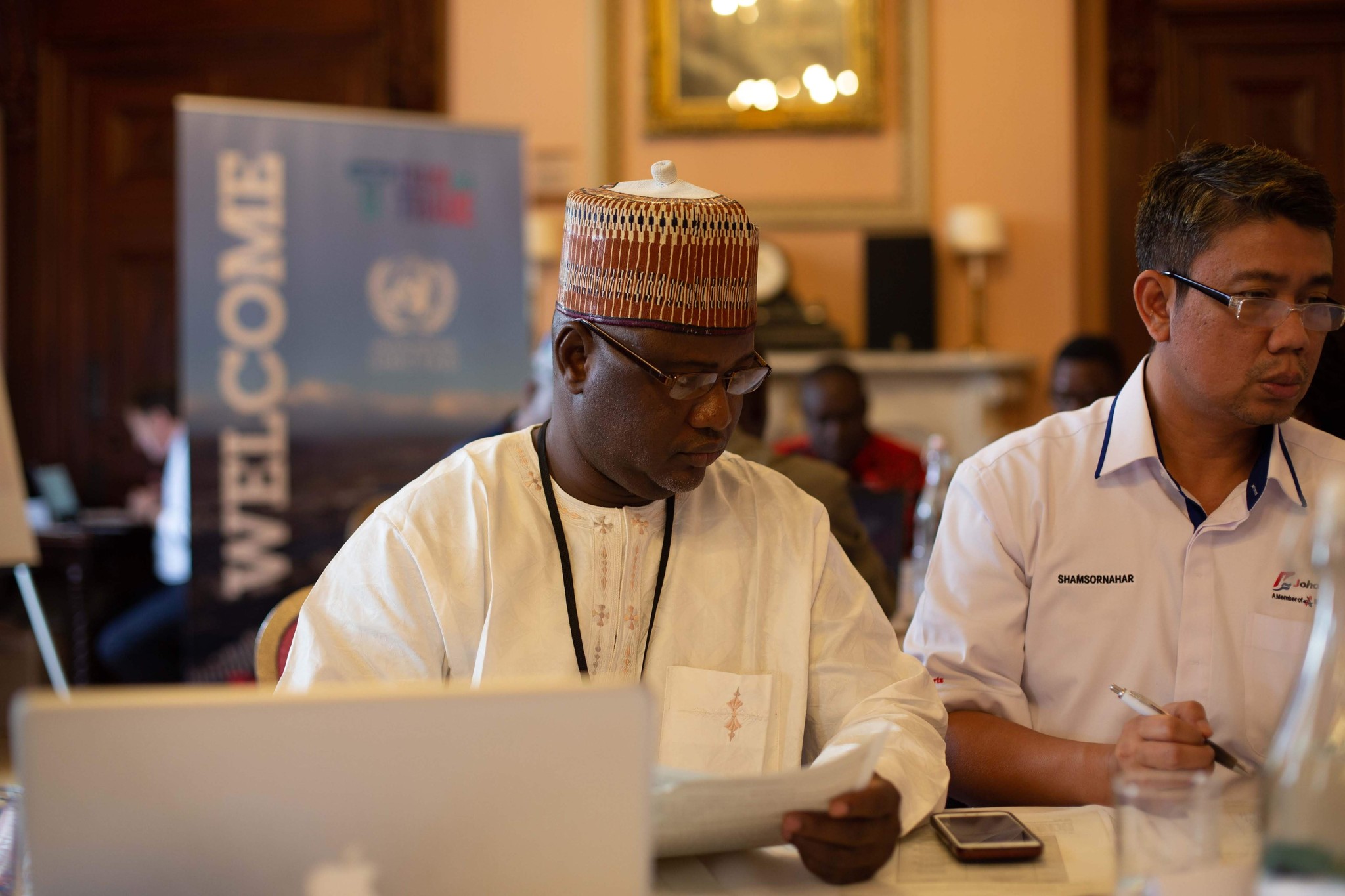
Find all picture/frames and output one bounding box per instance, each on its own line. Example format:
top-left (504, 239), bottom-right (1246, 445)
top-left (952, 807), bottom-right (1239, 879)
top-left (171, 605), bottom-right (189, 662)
top-left (590, 0), bottom-right (934, 229)
top-left (642, 0), bottom-right (897, 139)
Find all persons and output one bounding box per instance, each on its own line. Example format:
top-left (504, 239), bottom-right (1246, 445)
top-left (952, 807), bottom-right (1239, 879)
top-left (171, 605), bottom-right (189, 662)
top-left (1047, 331), bottom-right (1125, 414)
top-left (93, 381), bottom-right (275, 684)
top-left (770, 357), bottom-right (923, 577)
top-left (727, 341), bottom-right (897, 623)
top-left (900, 136), bottom-right (1345, 819)
top-left (270, 158), bottom-right (951, 886)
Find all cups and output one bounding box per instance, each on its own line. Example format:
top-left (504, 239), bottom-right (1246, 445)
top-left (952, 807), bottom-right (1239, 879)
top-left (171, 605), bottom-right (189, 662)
top-left (1112, 772), bottom-right (1262, 896)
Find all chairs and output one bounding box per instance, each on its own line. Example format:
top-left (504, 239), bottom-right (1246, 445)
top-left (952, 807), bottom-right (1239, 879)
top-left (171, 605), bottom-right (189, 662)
top-left (254, 584), bottom-right (314, 685)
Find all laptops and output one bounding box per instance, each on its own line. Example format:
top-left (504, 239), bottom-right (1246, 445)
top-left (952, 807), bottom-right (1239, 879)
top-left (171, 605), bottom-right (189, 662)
top-left (35, 464), bottom-right (135, 531)
top-left (15, 677), bottom-right (653, 896)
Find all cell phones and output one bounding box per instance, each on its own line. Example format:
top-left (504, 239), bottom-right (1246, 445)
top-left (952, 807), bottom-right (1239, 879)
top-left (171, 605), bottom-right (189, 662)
top-left (929, 811), bottom-right (1044, 863)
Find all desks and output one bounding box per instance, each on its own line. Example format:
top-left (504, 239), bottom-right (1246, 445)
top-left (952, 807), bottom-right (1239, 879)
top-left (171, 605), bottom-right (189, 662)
top-left (654, 805), bottom-right (1345, 896)
top-left (37, 515), bottom-right (147, 675)
top-left (763, 350), bottom-right (1040, 464)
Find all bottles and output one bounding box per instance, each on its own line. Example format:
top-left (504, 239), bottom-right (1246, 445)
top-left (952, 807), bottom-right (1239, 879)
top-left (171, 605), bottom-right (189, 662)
top-left (898, 436), bottom-right (956, 636)
top-left (1254, 475), bottom-right (1344, 896)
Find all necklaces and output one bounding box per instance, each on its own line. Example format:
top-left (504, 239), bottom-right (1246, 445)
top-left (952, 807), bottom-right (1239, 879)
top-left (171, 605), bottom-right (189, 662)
top-left (535, 416), bottom-right (678, 684)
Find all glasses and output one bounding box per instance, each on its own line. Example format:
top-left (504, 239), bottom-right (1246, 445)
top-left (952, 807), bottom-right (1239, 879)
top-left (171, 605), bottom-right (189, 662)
top-left (561, 317), bottom-right (773, 401)
top-left (1157, 271), bottom-right (1345, 332)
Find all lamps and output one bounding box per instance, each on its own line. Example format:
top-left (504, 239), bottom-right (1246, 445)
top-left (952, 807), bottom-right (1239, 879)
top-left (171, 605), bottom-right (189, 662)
top-left (948, 206), bottom-right (1008, 352)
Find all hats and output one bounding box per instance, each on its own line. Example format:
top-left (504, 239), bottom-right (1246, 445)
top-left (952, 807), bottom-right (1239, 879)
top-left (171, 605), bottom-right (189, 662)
top-left (557, 160), bottom-right (759, 337)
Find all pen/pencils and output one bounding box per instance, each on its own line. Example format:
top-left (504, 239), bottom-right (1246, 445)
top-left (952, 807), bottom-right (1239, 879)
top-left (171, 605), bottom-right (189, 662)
top-left (1108, 684), bottom-right (1248, 777)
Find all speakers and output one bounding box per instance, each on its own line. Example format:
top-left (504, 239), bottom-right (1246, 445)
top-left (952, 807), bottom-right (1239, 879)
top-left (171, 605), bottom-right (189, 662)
top-left (863, 232), bottom-right (936, 350)
